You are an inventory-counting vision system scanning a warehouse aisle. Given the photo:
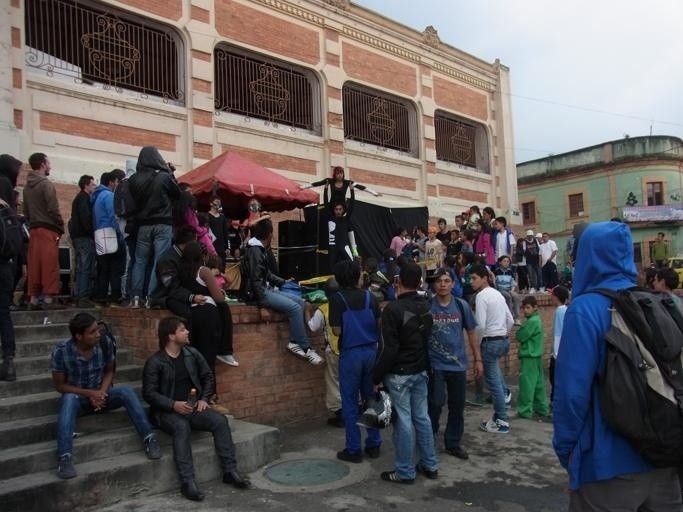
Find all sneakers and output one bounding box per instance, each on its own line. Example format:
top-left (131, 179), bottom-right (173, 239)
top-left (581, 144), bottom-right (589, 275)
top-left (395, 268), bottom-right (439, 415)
top-left (142, 295), bottom-right (152, 308)
top-left (381, 470), bottom-right (416, 484)
top-left (27, 302), bottom-right (41, 310)
top-left (127, 296), bottom-right (140, 309)
top-left (214, 354), bottom-right (239, 368)
top-left (222, 469), bottom-right (251, 489)
top-left (56, 453), bottom-right (77, 479)
top-left (364, 444), bottom-right (380, 458)
top-left (479, 418), bottom-right (510, 433)
top-left (77, 297), bottom-right (95, 308)
top-left (94, 299), bottom-right (110, 308)
top-left (304, 347), bottom-right (326, 366)
top-left (415, 461), bottom-right (438, 479)
top-left (337, 448), bottom-right (361, 464)
top-left (0, 357), bottom-right (16, 382)
top-left (445, 444), bottom-right (469, 460)
top-left (109, 299), bottom-right (126, 309)
top-left (41, 301), bottom-right (67, 310)
top-left (181, 478), bottom-right (204, 501)
top-left (284, 342), bottom-right (309, 361)
top-left (143, 433), bottom-right (162, 461)
top-left (326, 412), bottom-right (345, 428)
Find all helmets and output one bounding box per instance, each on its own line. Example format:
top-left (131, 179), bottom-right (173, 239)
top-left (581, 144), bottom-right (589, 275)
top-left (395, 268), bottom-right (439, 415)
top-left (355, 389), bottom-right (397, 430)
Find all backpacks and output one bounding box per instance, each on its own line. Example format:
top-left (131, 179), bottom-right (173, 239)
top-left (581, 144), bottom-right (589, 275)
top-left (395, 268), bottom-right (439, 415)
top-left (597, 283), bottom-right (683, 468)
top-left (0, 207), bottom-right (24, 260)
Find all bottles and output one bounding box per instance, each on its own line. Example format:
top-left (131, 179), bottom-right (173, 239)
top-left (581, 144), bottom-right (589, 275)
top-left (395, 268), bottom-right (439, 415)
top-left (187, 388), bottom-right (197, 406)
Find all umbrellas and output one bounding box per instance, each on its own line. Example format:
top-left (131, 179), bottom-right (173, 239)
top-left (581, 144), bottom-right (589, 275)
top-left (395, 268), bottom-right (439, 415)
top-left (177, 148), bottom-right (318, 210)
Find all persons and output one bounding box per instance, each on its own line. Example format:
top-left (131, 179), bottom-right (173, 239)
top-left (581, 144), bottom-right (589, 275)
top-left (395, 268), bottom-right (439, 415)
top-left (552, 222), bottom-right (683, 511)
top-left (468, 264), bottom-right (515, 434)
top-left (304, 279), bottom-right (345, 428)
top-left (165, 241), bottom-right (238, 367)
top-left (142, 317), bottom-right (252, 502)
top-left (239, 219), bottom-right (328, 370)
top-left (328, 259), bottom-right (379, 463)
top-left (240, 193), bottom-right (273, 257)
top-left (429, 268), bottom-right (484, 459)
top-left (12, 190), bottom-right (29, 310)
top-left (296, 167), bottom-right (382, 257)
top-left (324, 179), bottom-right (355, 274)
top-left (364, 206), bottom-right (580, 418)
top-left (373, 263), bottom-right (438, 484)
top-left (49, 313), bottom-right (162, 479)
top-left (67, 145), bottom-right (237, 310)
top-left (23, 153), bottom-right (64, 310)
top-left (1, 153), bottom-right (30, 381)
top-left (631, 232), bottom-right (682, 323)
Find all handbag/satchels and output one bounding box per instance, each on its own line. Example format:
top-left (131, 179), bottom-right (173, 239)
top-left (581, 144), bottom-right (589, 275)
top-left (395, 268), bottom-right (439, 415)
top-left (93, 226), bottom-right (119, 256)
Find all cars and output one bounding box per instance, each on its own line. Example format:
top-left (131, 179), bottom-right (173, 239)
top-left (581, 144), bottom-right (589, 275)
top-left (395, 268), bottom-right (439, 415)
top-left (665, 255), bottom-right (682, 289)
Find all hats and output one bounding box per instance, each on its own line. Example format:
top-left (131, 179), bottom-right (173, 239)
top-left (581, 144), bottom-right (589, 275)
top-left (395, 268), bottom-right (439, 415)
top-left (552, 285), bottom-right (569, 300)
top-left (535, 233), bottom-right (543, 239)
top-left (425, 268), bottom-right (452, 285)
top-left (525, 230), bottom-right (534, 237)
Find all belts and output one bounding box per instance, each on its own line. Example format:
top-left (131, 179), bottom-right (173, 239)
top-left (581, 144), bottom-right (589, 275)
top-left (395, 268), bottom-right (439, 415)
top-left (480, 334), bottom-right (511, 341)
